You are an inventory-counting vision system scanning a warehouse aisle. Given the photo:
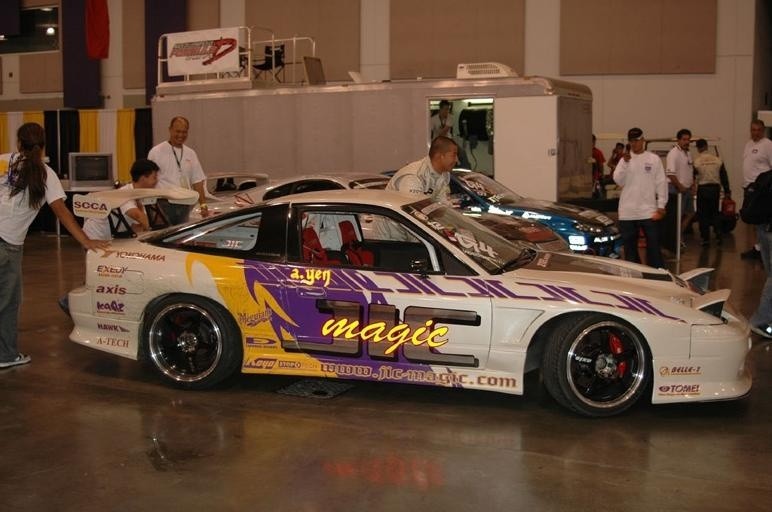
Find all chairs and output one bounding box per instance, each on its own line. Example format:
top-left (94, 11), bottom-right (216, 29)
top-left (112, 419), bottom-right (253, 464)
top-left (252, 44), bottom-right (285, 84)
top-left (300, 227), bottom-right (342, 266)
top-left (221, 45), bottom-right (249, 79)
top-left (338, 219), bottom-right (375, 268)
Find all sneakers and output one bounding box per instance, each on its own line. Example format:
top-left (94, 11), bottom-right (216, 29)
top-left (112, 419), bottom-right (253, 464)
top-left (0, 353), bottom-right (32, 367)
top-left (750, 323), bottom-right (772, 339)
top-left (699, 239), bottom-right (723, 246)
top-left (741, 247), bottom-right (761, 260)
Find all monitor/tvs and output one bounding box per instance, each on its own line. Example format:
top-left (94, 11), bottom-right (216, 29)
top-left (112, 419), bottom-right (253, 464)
top-left (69, 152), bottom-right (114, 187)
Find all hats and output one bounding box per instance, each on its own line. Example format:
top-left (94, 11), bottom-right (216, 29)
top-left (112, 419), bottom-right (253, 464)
top-left (628, 128), bottom-right (642, 139)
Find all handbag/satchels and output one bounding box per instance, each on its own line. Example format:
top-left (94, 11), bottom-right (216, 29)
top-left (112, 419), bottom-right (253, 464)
top-left (720, 198), bottom-right (739, 233)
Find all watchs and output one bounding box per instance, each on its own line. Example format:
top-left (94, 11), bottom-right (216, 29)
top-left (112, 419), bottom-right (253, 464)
top-left (200, 203), bottom-right (208, 209)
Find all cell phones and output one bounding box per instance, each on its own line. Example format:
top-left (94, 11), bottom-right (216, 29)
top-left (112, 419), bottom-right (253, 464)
top-left (625, 143), bottom-right (630, 154)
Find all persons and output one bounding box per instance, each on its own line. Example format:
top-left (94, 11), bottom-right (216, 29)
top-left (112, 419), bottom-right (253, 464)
top-left (431, 100), bottom-right (456, 141)
top-left (384, 136), bottom-right (459, 209)
top-left (592, 119), bottom-right (772, 339)
top-left (82, 159), bottom-right (160, 253)
top-left (146, 116), bottom-right (209, 231)
top-left (0, 122), bottom-right (110, 368)
